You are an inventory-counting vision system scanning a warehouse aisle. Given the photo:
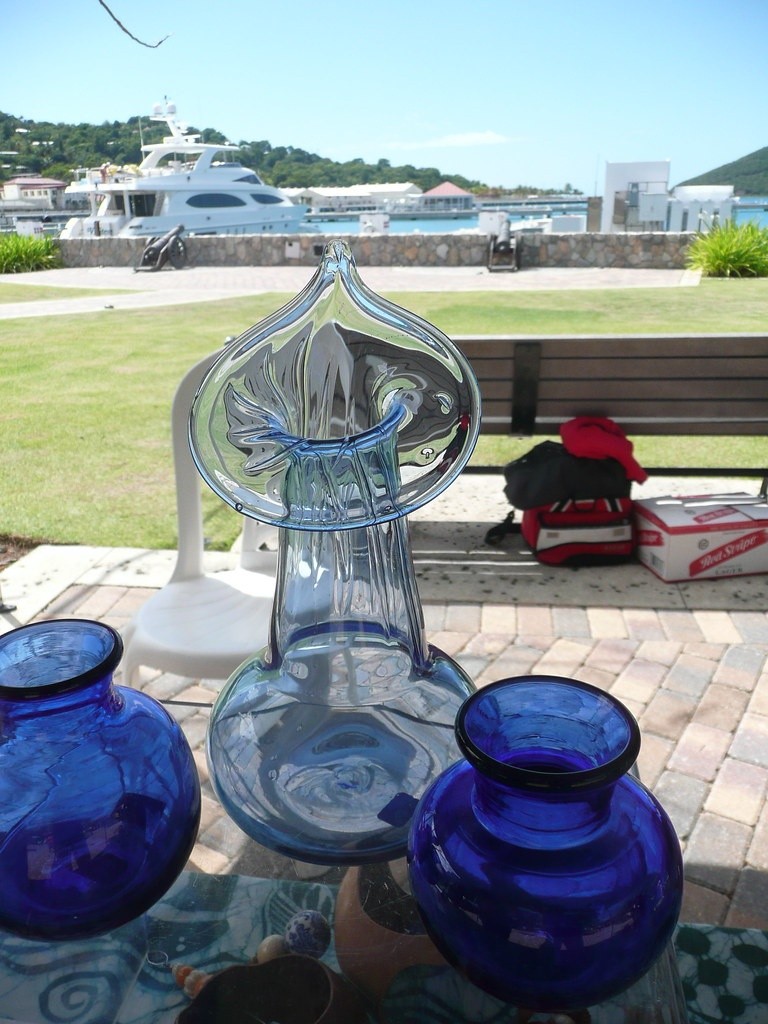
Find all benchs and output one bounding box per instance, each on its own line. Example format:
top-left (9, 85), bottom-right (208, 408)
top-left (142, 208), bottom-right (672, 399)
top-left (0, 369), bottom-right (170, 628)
top-left (449, 332), bottom-right (768, 495)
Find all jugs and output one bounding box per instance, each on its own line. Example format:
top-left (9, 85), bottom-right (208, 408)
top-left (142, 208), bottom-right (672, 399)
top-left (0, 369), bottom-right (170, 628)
top-left (183, 241), bottom-right (482, 866)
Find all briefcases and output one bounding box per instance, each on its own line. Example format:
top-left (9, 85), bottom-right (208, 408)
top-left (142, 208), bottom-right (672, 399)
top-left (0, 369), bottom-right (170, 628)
top-left (519, 496), bottom-right (636, 571)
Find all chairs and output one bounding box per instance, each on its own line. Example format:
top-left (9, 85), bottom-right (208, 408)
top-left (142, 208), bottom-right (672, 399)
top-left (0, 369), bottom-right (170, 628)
top-left (120, 349), bottom-right (281, 693)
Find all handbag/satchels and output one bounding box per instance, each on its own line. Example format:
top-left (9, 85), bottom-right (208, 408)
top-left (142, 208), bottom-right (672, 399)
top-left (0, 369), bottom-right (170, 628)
top-left (502, 440), bottom-right (631, 509)
top-left (560, 412), bottom-right (647, 483)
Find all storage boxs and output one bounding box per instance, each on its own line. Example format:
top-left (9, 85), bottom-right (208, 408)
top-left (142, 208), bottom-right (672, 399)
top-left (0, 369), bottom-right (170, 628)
top-left (632, 492), bottom-right (768, 584)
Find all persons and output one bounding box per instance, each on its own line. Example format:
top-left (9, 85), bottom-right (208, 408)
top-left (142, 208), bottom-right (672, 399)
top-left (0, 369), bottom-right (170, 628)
top-left (100, 161), bottom-right (110, 183)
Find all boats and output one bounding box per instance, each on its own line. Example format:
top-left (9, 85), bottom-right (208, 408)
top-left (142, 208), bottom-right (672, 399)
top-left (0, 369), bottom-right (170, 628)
top-left (59, 96), bottom-right (321, 240)
